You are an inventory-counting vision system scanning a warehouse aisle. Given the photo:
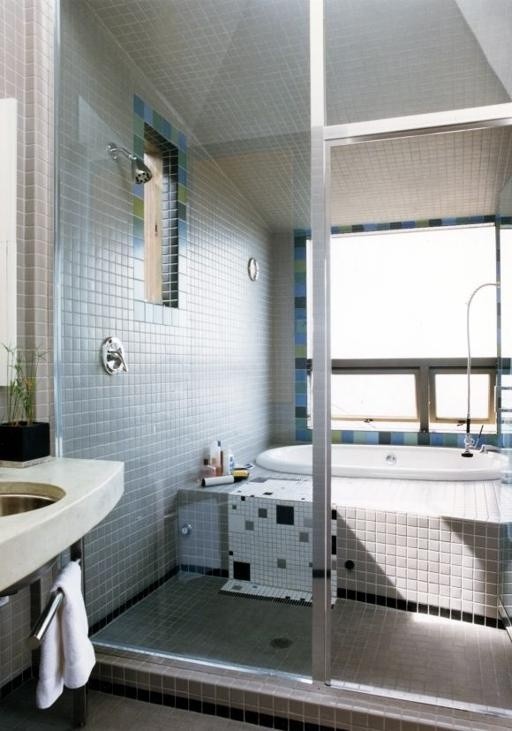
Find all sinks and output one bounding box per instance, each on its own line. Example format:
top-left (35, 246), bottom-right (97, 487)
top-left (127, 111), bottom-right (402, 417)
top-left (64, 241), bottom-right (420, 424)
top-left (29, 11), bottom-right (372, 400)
top-left (0, 479), bottom-right (67, 518)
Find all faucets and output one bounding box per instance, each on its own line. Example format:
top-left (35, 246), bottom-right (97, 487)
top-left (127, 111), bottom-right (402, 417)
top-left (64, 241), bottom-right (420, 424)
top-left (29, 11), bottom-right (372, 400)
top-left (479, 445), bottom-right (498, 454)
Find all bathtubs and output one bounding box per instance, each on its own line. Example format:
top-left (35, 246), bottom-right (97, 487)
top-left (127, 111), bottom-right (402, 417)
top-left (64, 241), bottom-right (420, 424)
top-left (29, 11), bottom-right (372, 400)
top-left (255, 442), bottom-right (511, 483)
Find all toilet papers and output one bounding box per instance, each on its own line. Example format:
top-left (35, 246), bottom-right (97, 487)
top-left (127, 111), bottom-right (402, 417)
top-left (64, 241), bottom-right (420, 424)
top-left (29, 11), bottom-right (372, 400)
top-left (204, 476), bottom-right (236, 486)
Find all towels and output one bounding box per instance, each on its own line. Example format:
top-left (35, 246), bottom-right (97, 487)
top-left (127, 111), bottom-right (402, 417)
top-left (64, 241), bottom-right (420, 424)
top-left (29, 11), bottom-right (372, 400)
top-left (36, 561), bottom-right (95, 709)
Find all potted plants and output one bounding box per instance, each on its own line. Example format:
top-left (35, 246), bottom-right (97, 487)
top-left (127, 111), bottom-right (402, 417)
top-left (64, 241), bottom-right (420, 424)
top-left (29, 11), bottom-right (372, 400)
top-left (1, 343), bottom-right (50, 461)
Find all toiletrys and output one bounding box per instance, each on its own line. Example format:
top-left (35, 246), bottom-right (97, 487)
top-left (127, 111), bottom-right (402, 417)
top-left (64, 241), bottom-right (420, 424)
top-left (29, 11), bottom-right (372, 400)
top-left (209, 440), bottom-right (234, 475)
